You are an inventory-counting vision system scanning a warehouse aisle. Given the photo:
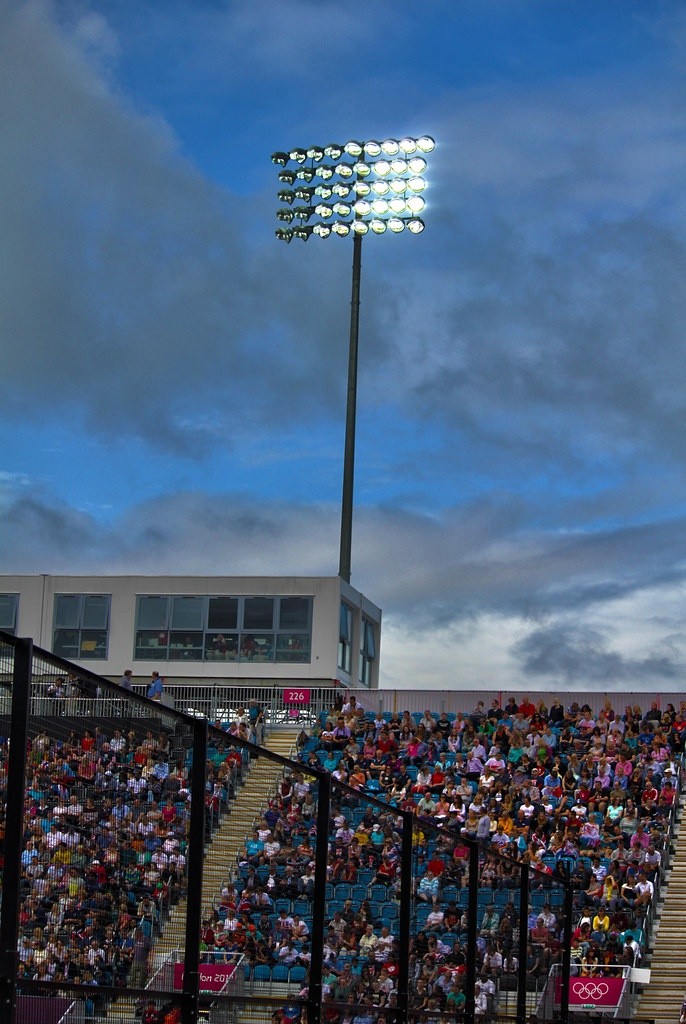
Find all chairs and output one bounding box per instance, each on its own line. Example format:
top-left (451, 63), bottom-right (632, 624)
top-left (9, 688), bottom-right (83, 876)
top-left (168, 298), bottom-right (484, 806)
top-left (17, 711), bottom-right (682, 1020)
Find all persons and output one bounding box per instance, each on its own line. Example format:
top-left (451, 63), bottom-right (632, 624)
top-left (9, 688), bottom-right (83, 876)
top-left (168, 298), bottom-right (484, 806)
top-left (0, 635), bottom-right (686, 1024)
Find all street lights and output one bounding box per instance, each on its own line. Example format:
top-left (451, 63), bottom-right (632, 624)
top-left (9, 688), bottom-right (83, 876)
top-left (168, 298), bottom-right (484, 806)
top-left (272, 138), bottom-right (437, 583)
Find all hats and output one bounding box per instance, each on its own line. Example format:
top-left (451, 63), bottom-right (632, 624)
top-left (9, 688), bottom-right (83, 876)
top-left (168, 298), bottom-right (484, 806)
top-left (76, 845), bottom-right (84, 850)
top-left (664, 769), bottom-right (671, 772)
top-left (456, 712), bottom-right (463, 717)
top-left (532, 768), bottom-right (537, 772)
top-left (515, 766), bottom-right (526, 773)
top-left (576, 799), bottom-right (582, 804)
top-left (90, 860), bottom-right (100, 866)
top-left (166, 832), bottom-right (174, 835)
